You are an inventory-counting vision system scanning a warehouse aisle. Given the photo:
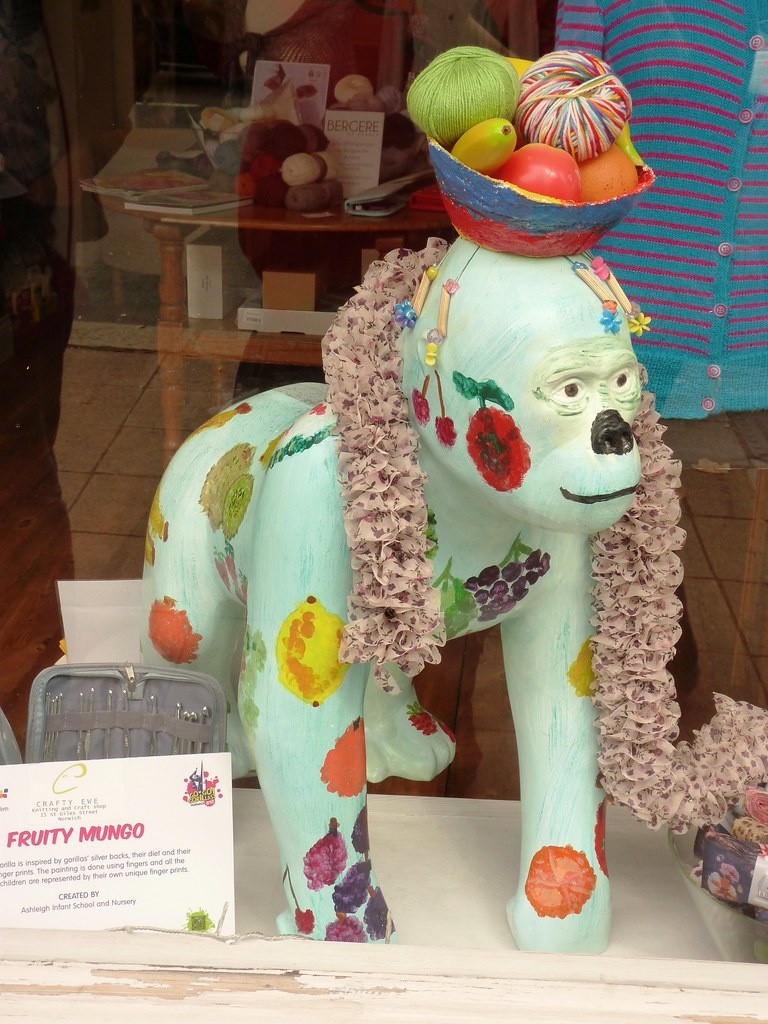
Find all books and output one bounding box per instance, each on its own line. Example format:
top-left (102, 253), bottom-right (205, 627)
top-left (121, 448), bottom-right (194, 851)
top-left (79, 168), bottom-right (209, 201)
top-left (124, 186), bottom-right (254, 215)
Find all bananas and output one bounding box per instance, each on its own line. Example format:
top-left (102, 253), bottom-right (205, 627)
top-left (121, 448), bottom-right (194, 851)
top-left (452, 118), bottom-right (517, 174)
top-left (508, 57), bottom-right (646, 172)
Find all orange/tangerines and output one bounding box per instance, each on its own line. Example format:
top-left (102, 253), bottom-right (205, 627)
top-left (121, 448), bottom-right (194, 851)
top-left (580, 146), bottom-right (639, 202)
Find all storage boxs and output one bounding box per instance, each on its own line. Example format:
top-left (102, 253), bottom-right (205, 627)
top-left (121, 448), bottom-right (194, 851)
top-left (185, 231), bottom-right (421, 336)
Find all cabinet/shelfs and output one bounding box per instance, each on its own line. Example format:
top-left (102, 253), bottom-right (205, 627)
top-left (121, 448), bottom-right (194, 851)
top-left (95, 191), bottom-right (452, 470)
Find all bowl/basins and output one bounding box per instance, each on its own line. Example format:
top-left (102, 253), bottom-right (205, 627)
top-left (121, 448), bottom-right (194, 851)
top-left (424, 131), bottom-right (655, 257)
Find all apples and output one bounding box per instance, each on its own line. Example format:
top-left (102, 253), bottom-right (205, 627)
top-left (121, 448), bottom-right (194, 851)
top-left (494, 142), bottom-right (583, 204)
